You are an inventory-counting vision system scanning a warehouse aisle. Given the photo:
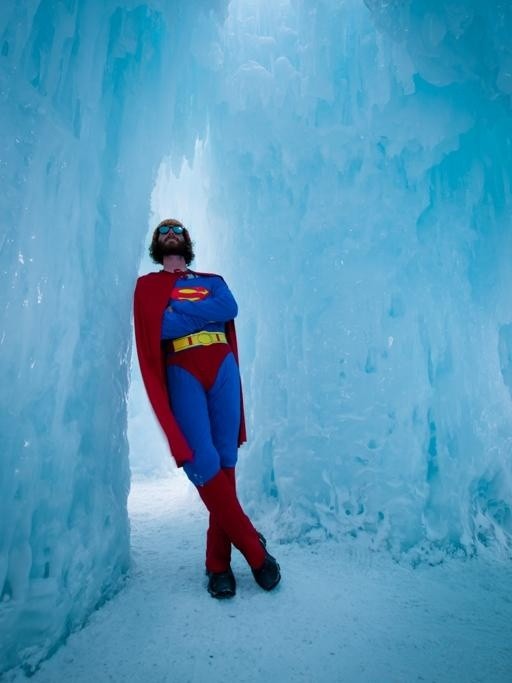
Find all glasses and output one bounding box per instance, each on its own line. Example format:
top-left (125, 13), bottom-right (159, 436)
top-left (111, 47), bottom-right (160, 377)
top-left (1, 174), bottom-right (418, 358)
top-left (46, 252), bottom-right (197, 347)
top-left (158, 225), bottom-right (183, 235)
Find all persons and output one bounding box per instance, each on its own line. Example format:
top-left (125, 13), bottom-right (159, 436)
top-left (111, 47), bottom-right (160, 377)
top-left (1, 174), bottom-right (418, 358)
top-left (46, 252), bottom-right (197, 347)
top-left (134, 220), bottom-right (281, 598)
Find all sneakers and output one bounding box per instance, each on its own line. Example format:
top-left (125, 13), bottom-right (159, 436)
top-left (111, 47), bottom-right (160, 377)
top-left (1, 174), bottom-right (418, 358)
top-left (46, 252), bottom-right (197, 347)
top-left (252, 532), bottom-right (280, 591)
top-left (206, 568), bottom-right (235, 599)
